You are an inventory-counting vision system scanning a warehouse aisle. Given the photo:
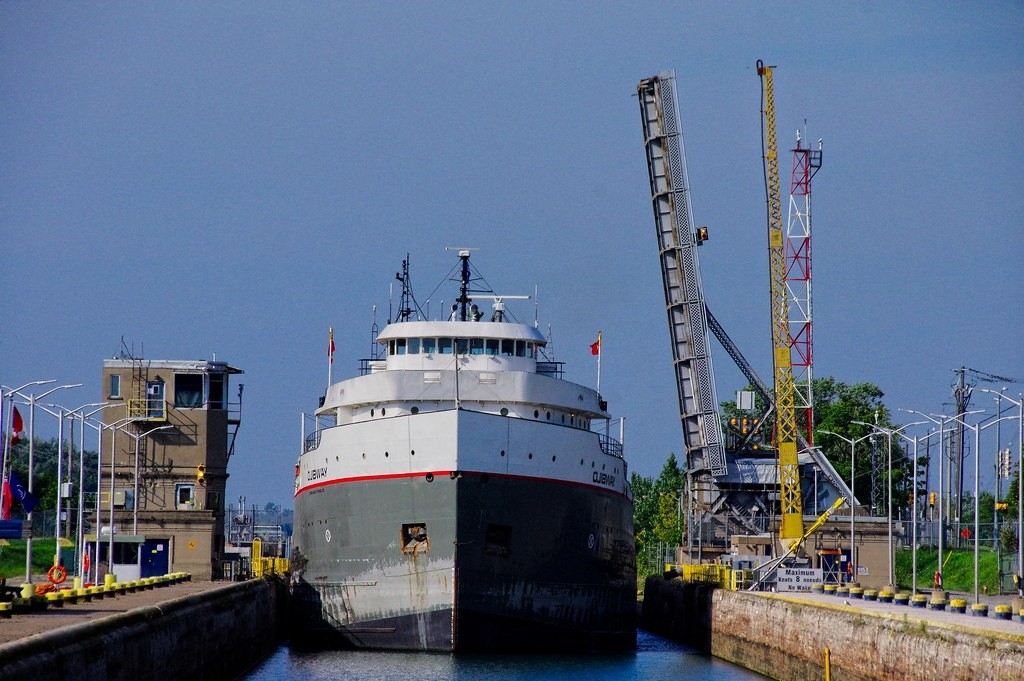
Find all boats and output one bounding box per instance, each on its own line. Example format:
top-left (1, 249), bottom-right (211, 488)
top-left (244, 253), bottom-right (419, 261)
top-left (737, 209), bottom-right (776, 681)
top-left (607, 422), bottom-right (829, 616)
top-left (284, 243), bottom-right (641, 660)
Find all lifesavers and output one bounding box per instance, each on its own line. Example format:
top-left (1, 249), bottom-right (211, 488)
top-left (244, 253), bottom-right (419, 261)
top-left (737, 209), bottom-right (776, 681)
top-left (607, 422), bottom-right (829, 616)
top-left (84, 555), bottom-right (89, 573)
top-left (847, 562), bottom-right (852, 575)
top-left (48, 566), bottom-right (67, 584)
top-left (934, 571), bottom-right (940, 589)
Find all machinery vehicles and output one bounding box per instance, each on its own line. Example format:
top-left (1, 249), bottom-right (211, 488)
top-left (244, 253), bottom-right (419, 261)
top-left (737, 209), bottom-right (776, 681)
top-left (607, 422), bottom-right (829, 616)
top-left (631, 55), bottom-right (854, 595)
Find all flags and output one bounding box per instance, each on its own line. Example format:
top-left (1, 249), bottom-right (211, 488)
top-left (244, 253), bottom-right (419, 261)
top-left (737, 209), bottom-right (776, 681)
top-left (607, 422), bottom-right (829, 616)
top-left (12, 405), bottom-right (23, 433)
top-left (590, 330), bottom-right (603, 355)
top-left (9, 470), bottom-right (40, 513)
top-left (328, 328), bottom-right (335, 364)
top-left (3, 482), bottom-right (12, 520)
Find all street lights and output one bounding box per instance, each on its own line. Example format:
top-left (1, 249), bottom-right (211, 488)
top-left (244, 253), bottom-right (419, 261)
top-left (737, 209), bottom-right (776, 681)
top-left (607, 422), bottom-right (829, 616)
top-left (46, 403), bottom-right (125, 576)
top-left (69, 416), bottom-right (136, 586)
top-left (980, 389), bottom-right (1024, 597)
top-left (897, 409), bottom-right (988, 590)
top-left (929, 411), bottom-right (1021, 604)
top-left (0, 377), bottom-right (57, 464)
top-left (849, 419), bottom-right (930, 587)
top-left (0, 383), bottom-right (84, 586)
top-left (817, 429), bottom-right (885, 583)
top-left (883, 427), bottom-right (959, 596)
top-left (85, 417), bottom-right (142, 575)
top-left (110, 425), bottom-right (175, 535)
top-left (35, 402), bottom-right (111, 589)
